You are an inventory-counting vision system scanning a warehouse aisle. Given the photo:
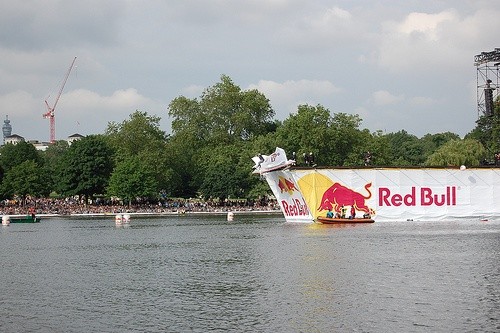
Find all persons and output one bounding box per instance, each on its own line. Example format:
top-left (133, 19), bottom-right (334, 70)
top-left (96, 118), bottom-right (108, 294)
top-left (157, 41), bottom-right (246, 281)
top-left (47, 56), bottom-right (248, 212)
top-left (334, 212), bottom-right (340, 219)
top-left (363, 210), bottom-right (370, 219)
top-left (364, 152), bottom-right (372, 166)
top-left (340, 206), bottom-right (346, 219)
top-left (350, 205), bottom-right (355, 220)
top-left (326, 209), bottom-right (333, 218)
top-left (302, 152), bottom-right (318, 168)
top-left (291, 152), bottom-right (297, 167)
top-left (0, 194), bottom-right (282, 216)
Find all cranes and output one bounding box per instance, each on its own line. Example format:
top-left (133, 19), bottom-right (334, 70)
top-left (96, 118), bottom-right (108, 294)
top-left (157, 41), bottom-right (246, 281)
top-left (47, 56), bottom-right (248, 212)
top-left (42, 56), bottom-right (77, 143)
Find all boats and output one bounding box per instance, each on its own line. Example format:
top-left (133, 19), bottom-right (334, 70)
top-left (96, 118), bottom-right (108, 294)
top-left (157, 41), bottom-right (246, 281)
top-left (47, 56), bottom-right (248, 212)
top-left (316, 215), bottom-right (375, 224)
top-left (250, 148), bottom-right (500, 223)
top-left (0, 213), bottom-right (42, 224)
